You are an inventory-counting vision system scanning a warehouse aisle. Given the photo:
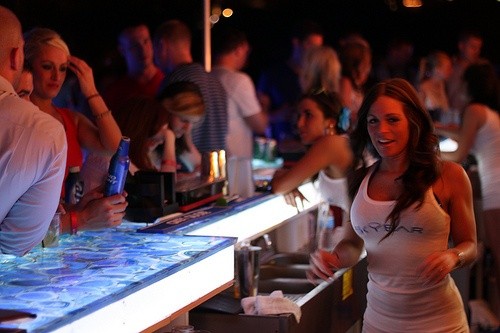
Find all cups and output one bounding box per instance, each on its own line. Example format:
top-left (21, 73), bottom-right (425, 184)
top-left (234, 244), bottom-right (262, 299)
top-left (43, 212), bottom-right (59, 248)
top-left (264, 140), bottom-right (276, 162)
top-left (253, 138), bottom-right (266, 160)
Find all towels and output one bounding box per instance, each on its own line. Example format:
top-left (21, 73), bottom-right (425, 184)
top-left (240, 290), bottom-right (302, 325)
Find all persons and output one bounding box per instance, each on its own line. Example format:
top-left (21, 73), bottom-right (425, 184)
top-left (307, 78), bottom-right (480, 333)
top-left (99, 18), bottom-right (496, 199)
top-left (272, 87), bottom-right (377, 250)
top-left (0, 5), bottom-right (67, 257)
top-left (13, 28), bottom-right (128, 236)
top-left (439, 64), bottom-right (500, 333)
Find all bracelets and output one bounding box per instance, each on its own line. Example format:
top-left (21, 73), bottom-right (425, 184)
top-left (93, 110), bottom-right (111, 121)
top-left (448, 248), bottom-right (465, 269)
top-left (86, 93), bottom-right (100, 101)
top-left (71, 211), bottom-right (77, 235)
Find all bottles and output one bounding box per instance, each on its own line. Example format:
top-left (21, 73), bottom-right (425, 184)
top-left (64, 166), bottom-right (84, 234)
top-left (104, 138), bottom-right (130, 197)
top-left (318, 206), bottom-right (334, 252)
